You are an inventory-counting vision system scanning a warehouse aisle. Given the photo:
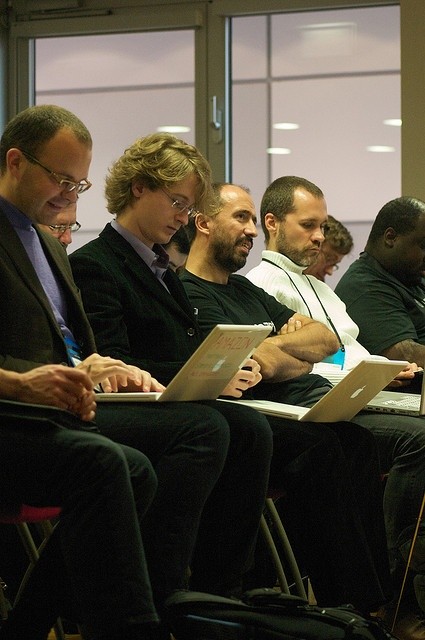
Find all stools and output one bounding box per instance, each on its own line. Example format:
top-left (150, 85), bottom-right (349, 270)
top-left (0, 504), bottom-right (65, 640)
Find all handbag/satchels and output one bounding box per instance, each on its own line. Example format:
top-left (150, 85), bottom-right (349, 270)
top-left (157, 586), bottom-right (398, 638)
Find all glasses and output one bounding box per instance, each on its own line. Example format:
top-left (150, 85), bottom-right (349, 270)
top-left (321, 250), bottom-right (339, 271)
top-left (158, 185), bottom-right (200, 218)
top-left (47, 220), bottom-right (81, 233)
top-left (22, 150), bottom-right (93, 194)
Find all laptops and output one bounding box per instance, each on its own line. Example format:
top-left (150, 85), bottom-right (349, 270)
top-left (216, 360), bottom-right (411, 423)
top-left (361, 362), bottom-right (425, 417)
top-left (96, 323), bottom-right (274, 403)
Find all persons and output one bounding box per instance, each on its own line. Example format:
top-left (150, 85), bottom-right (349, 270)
top-left (303, 215), bottom-right (353, 281)
top-left (175, 180), bottom-right (424, 638)
top-left (0, 103), bottom-right (273, 608)
top-left (66, 132), bottom-right (390, 628)
top-left (35, 200), bottom-right (82, 251)
top-left (334, 195), bottom-right (425, 365)
top-left (156, 224), bottom-right (191, 274)
top-left (1, 362), bottom-right (159, 640)
top-left (244, 175), bottom-right (424, 639)
